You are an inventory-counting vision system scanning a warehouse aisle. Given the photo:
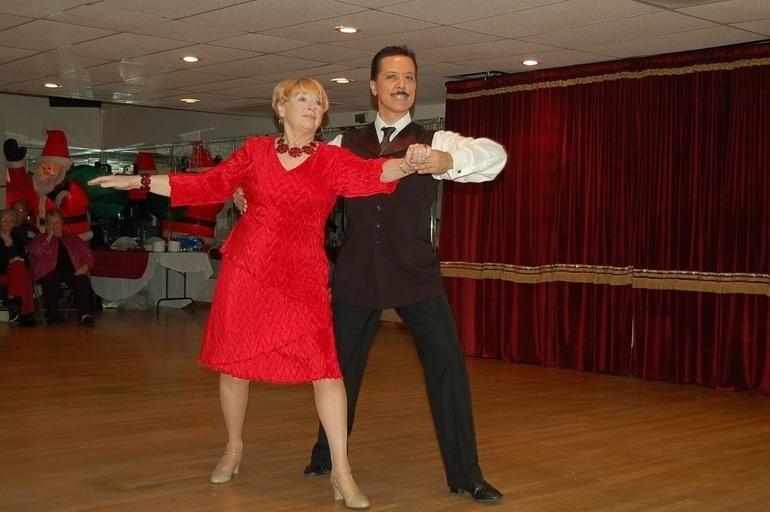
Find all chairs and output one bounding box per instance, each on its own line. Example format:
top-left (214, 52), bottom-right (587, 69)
top-left (25, 245), bottom-right (79, 328)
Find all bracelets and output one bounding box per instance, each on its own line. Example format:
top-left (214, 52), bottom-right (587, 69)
top-left (138, 173), bottom-right (152, 193)
top-left (399, 159), bottom-right (416, 174)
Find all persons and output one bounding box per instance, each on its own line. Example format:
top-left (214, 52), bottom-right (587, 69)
top-left (231, 47), bottom-right (509, 503)
top-left (87, 77), bottom-right (431, 510)
top-left (0, 200), bottom-right (96, 328)
top-left (3, 129), bottom-right (93, 243)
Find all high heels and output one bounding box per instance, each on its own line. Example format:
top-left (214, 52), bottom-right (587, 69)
top-left (329, 467), bottom-right (371, 510)
top-left (211, 441), bottom-right (244, 486)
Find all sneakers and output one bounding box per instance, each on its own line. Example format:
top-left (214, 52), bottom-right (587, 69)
top-left (6, 302), bottom-right (18, 322)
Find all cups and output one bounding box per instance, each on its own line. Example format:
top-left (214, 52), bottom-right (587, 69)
top-left (168, 240), bottom-right (181, 252)
top-left (152, 240), bottom-right (165, 252)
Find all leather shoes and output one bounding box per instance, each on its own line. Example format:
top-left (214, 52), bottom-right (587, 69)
top-left (447, 474), bottom-right (499, 502)
top-left (304, 461), bottom-right (329, 477)
top-left (80, 314), bottom-right (95, 328)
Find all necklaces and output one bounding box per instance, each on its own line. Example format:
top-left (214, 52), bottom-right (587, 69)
top-left (275, 132), bottom-right (318, 157)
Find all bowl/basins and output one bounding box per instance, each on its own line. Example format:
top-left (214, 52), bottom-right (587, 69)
top-left (143, 245), bottom-right (153, 251)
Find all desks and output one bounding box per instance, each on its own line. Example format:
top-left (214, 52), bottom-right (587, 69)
top-left (90, 250), bottom-right (215, 316)
